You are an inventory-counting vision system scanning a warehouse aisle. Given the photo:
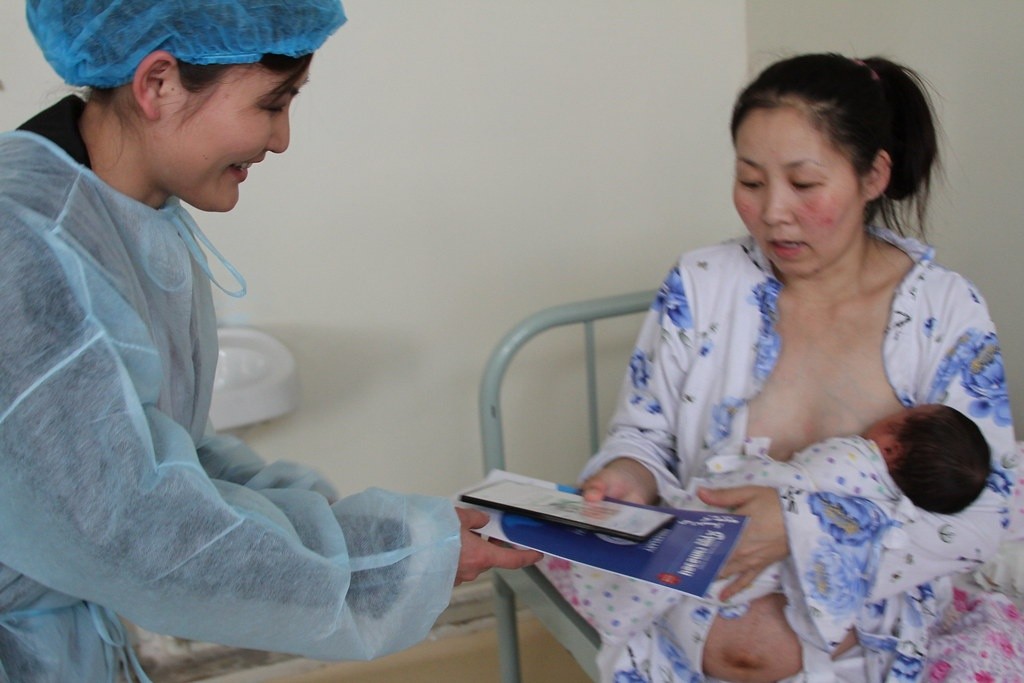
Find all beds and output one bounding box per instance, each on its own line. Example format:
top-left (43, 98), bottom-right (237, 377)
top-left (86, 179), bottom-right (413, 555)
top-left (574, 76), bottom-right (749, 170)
top-left (475, 287), bottom-right (1024, 683)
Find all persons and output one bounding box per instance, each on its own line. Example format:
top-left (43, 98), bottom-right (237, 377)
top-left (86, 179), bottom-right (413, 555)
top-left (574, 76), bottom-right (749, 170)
top-left (576, 51), bottom-right (1024, 683)
top-left (0, 1), bottom-right (547, 683)
top-left (664, 402), bottom-right (993, 605)
